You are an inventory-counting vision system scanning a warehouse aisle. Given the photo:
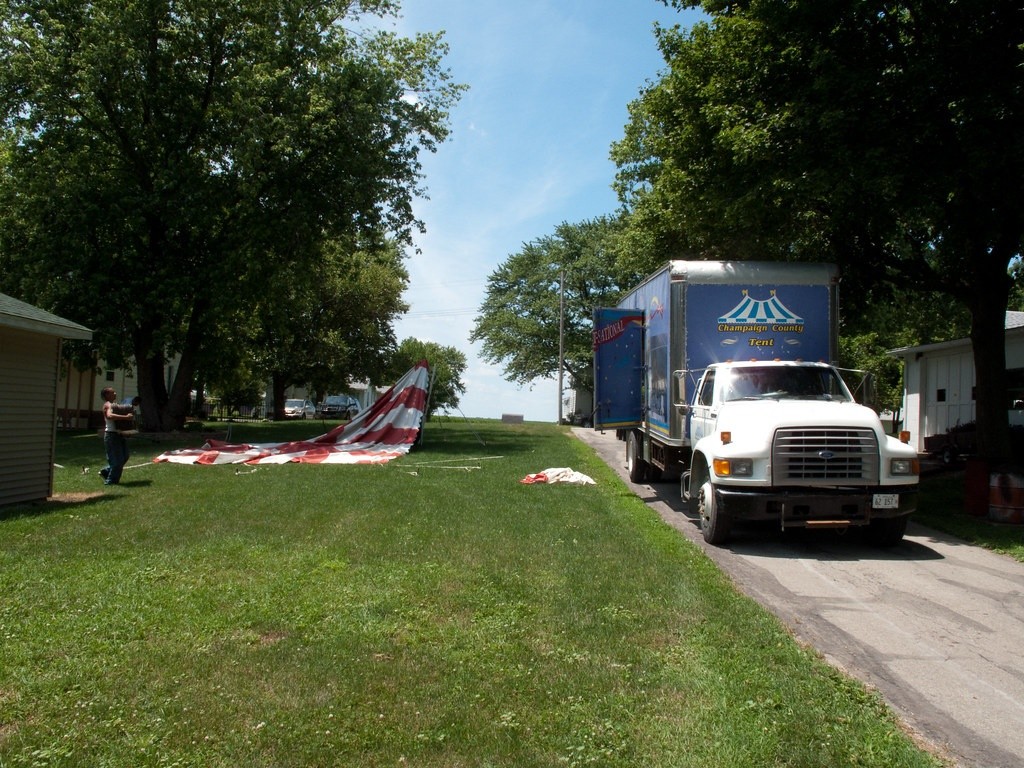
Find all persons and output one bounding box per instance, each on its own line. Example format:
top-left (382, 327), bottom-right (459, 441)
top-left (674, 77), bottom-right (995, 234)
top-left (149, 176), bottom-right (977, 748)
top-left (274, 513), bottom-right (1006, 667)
top-left (101, 386), bottom-right (142, 485)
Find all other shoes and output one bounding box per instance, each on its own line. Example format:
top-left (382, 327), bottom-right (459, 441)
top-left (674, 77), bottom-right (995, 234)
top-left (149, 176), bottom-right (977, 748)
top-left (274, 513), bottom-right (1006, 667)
top-left (98, 471), bottom-right (107, 481)
top-left (104, 482), bottom-right (109, 485)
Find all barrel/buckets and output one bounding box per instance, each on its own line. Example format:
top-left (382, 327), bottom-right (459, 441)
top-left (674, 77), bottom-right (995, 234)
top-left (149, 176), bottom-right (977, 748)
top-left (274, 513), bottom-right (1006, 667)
top-left (988, 465), bottom-right (1024, 523)
top-left (962, 454), bottom-right (989, 514)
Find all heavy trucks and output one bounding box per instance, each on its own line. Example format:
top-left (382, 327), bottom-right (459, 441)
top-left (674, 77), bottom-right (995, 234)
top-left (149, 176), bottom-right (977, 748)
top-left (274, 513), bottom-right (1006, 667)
top-left (592, 259), bottom-right (922, 548)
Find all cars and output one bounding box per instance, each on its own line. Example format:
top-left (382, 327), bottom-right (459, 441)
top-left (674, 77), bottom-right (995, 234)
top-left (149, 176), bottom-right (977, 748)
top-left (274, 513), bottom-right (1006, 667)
top-left (284, 398), bottom-right (316, 420)
top-left (313, 395), bottom-right (361, 421)
